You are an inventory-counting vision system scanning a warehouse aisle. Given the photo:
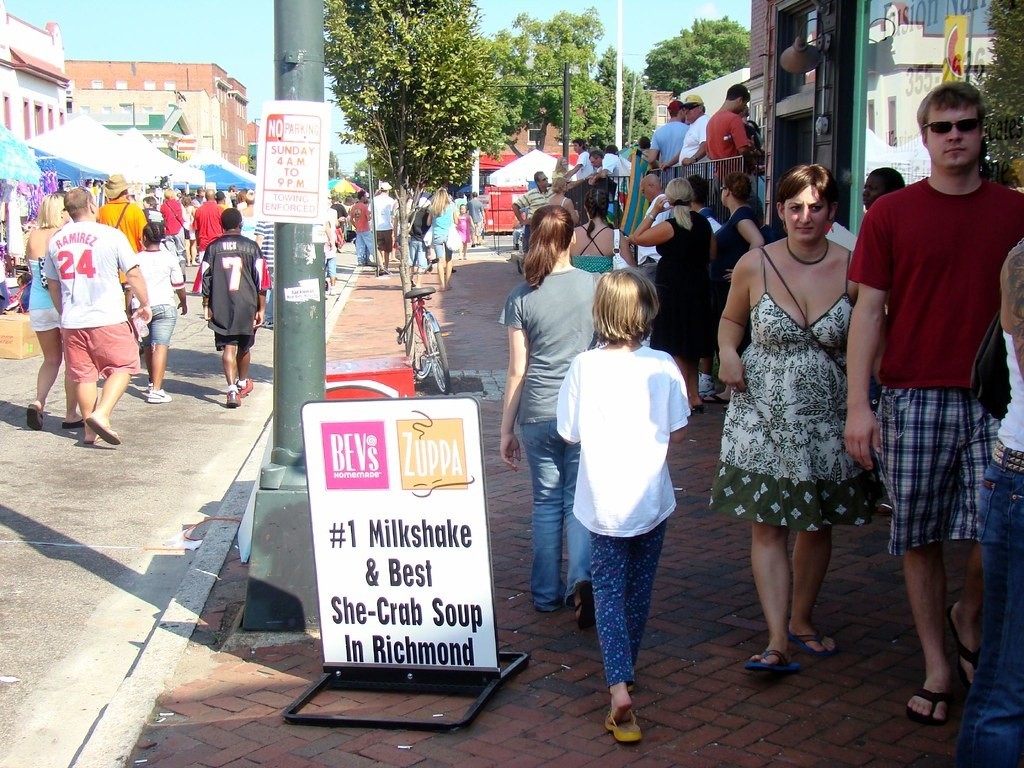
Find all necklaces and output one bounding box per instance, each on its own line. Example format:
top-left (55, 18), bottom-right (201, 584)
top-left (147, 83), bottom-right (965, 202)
top-left (787, 236), bottom-right (829, 265)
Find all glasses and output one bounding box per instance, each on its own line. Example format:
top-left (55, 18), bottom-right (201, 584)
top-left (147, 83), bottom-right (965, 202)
top-left (540, 177), bottom-right (548, 181)
top-left (922, 118), bottom-right (980, 134)
top-left (719, 187), bottom-right (732, 194)
top-left (685, 104), bottom-right (700, 108)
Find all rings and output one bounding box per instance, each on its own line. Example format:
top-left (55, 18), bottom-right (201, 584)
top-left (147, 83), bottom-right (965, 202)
top-left (731, 387), bottom-right (737, 390)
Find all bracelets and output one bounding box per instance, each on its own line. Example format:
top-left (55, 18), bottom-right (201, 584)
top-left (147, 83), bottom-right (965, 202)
top-left (649, 214), bottom-right (656, 221)
top-left (202, 302), bottom-right (209, 306)
top-left (692, 156), bottom-right (698, 162)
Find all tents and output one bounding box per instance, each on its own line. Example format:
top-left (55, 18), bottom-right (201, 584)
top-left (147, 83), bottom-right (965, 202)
top-left (486, 148), bottom-right (578, 255)
top-left (0, 116), bottom-right (256, 188)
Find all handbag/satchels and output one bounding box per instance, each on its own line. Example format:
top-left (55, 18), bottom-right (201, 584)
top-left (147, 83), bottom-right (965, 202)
top-left (446, 223), bottom-right (461, 251)
top-left (183, 229), bottom-right (191, 240)
top-left (612, 229), bottom-right (631, 270)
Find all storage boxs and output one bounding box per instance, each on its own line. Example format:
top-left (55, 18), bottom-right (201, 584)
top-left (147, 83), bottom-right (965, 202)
top-left (0, 313), bottom-right (40, 360)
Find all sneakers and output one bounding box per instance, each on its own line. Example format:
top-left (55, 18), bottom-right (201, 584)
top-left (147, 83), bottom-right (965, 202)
top-left (148, 383), bottom-right (172, 403)
top-left (237, 379), bottom-right (253, 397)
top-left (226, 391), bottom-right (241, 408)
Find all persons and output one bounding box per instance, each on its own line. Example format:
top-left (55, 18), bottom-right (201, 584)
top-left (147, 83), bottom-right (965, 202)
top-left (500, 205), bottom-right (601, 627)
top-left (863, 167), bottom-right (905, 210)
top-left (717, 162), bottom-right (882, 671)
top-left (94, 175), bottom-right (280, 407)
top-left (41, 185), bottom-right (152, 446)
top-left (26, 191), bottom-right (85, 429)
top-left (0, 259), bottom-right (32, 312)
top-left (846, 81), bottom-right (1024, 717)
top-left (325, 182), bottom-right (487, 296)
top-left (512, 84), bottom-right (779, 412)
top-left (954, 237), bottom-right (1024, 768)
top-left (556, 271), bottom-right (692, 742)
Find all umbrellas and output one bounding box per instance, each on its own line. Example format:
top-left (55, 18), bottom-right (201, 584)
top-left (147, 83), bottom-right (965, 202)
top-left (328, 178), bottom-right (369, 198)
top-left (458, 185), bottom-right (484, 194)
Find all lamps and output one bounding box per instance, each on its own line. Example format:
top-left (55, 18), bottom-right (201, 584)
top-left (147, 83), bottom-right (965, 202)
top-left (779, 18), bottom-right (833, 76)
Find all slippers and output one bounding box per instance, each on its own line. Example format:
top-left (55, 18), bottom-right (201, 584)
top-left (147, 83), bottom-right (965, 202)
top-left (906, 688), bottom-right (951, 726)
top-left (626, 682), bottom-right (635, 691)
top-left (744, 650), bottom-right (800, 671)
top-left (605, 708), bottom-right (642, 741)
top-left (574, 582), bottom-right (598, 629)
top-left (61, 419), bottom-right (84, 429)
top-left (84, 416), bottom-right (120, 445)
top-left (702, 393), bottom-right (730, 403)
top-left (788, 630), bottom-right (836, 655)
top-left (946, 605), bottom-right (982, 689)
top-left (690, 403), bottom-right (704, 413)
top-left (27, 404), bottom-right (44, 430)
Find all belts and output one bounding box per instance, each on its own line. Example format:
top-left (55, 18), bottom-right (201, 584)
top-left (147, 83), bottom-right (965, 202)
top-left (992, 442), bottom-right (1024, 473)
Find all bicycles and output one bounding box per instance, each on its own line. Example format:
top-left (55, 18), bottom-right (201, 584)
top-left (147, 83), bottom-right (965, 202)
top-left (393, 256), bottom-right (453, 396)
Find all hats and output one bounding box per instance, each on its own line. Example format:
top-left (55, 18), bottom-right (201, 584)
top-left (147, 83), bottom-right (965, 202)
top-left (380, 181), bottom-right (392, 191)
top-left (104, 174), bottom-right (131, 199)
top-left (667, 101), bottom-right (682, 110)
top-left (679, 95), bottom-right (705, 109)
